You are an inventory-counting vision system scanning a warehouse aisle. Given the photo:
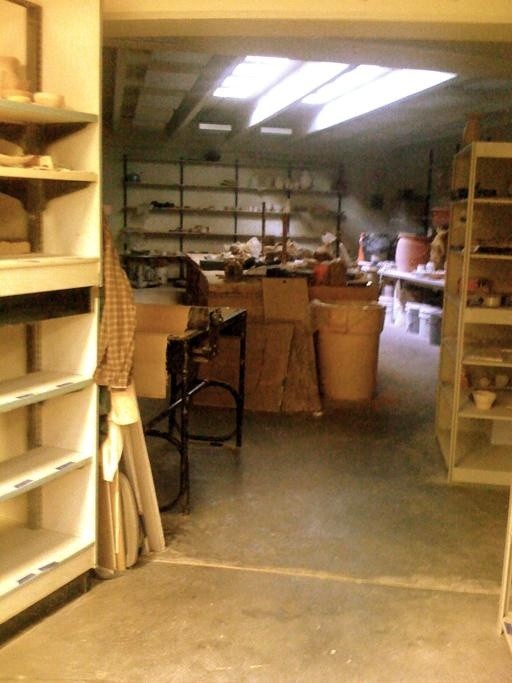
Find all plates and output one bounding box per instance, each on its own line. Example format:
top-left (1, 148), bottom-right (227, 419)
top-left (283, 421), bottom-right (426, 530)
top-left (0, 154), bottom-right (32, 166)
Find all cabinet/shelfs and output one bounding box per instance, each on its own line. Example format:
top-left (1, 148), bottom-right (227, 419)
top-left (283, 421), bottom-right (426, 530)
top-left (118, 153), bottom-right (347, 258)
top-left (0, 0), bottom-right (103, 648)
top-left (435, 141), bottom-right (512, 486)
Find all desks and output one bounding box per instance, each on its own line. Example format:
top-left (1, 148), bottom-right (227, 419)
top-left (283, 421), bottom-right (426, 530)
top-left (135, 307), bottom-right (247, 515)
top-left (361, 267), bottom-right (445, 327)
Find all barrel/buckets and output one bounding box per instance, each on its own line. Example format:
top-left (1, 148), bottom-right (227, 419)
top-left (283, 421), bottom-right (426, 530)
top-left (312, 294), bottom-right (386, 407)
top-left (396, 229), bottom-right (432, 274)
top-left (403, 300), bottom-right (428, 334)
top-left (416, 306), bottom-right (443, 344)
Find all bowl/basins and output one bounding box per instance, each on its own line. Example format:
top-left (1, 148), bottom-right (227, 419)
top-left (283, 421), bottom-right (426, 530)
top-left (472, 390), bottom-right (497, 410)
top-left (34, 92), bottom-right (65, 106)
